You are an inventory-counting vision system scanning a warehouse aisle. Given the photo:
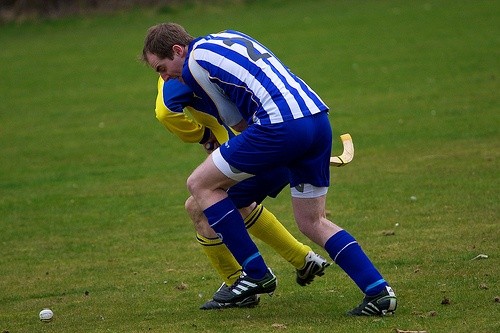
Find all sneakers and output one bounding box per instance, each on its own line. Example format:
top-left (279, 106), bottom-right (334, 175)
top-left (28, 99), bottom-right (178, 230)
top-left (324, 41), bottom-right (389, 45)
top-left (296, 250), bottom-right (330, 286)
top-left (200, 282), bottom-right (261, 308)
top-left (213, 266), bottom-right (275, 306)
top-left (348, 285), bottom-right (398, 316)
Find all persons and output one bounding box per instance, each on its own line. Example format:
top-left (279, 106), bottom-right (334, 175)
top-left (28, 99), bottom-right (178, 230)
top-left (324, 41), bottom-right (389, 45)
top-left (140, 20), bottom-right (398, 315)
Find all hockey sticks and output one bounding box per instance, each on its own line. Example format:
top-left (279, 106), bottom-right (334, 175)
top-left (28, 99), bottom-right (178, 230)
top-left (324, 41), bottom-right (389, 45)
top-left (205, 133), bottom-right (355, 167)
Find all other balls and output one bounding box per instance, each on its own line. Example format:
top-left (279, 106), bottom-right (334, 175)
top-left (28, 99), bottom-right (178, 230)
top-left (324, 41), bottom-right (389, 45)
top-left (39, 308), bottom-right (54, 323)
